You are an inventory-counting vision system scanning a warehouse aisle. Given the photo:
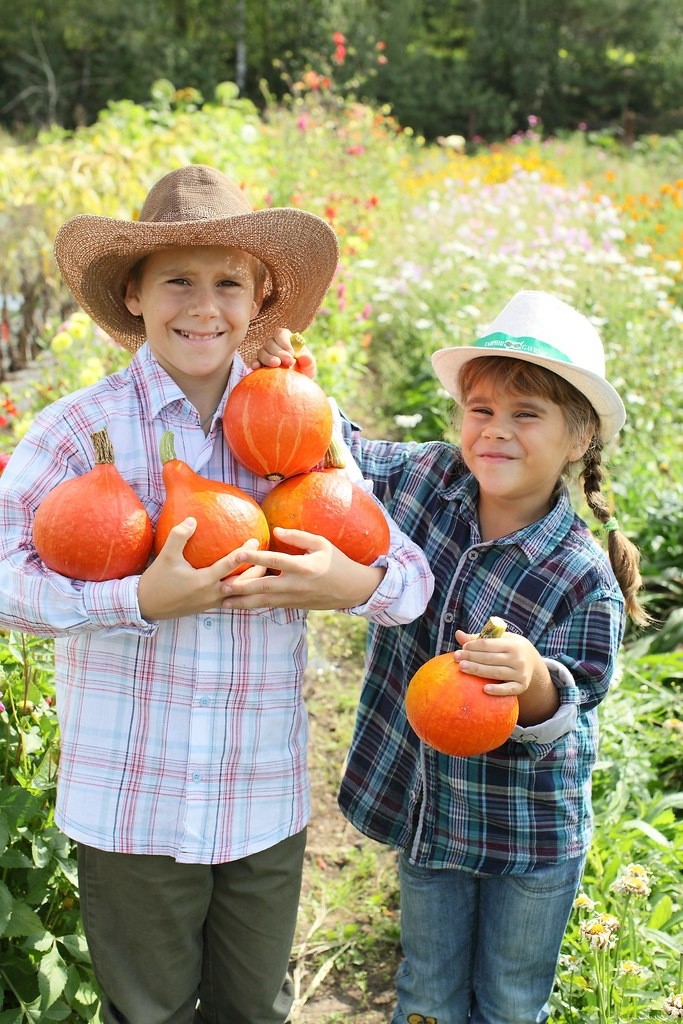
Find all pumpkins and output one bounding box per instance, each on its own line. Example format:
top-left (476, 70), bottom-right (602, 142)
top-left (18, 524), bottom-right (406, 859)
top-left (405, 651), bottom-right (519, 756)
top-left (32, 369), bottom-right (391, 578)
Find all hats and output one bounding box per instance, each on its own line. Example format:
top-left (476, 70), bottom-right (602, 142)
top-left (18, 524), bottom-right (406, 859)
top-left (432, 291), bottom-right (626, 444)
top-left (55, 165), bottom-right (339, 369)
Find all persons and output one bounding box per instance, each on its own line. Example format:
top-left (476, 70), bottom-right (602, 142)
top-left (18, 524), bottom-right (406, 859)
top-left (0, 166), bottom-right (433, 1024)
top-left (250, 291), bottom-right (655, 1023)
